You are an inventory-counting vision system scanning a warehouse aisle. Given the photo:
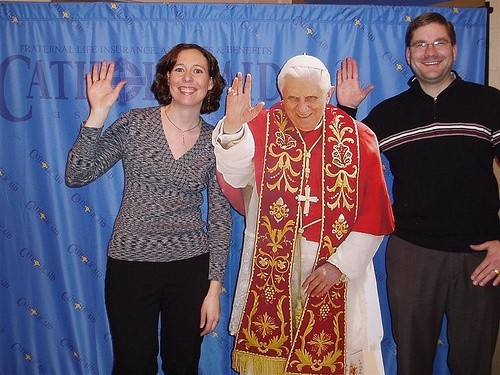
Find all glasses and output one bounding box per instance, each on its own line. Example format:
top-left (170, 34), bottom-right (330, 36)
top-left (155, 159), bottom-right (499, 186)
top-left (411, 40), bottom-right (452, 51)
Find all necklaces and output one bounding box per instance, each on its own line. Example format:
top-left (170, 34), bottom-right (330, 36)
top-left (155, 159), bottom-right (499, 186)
top-left (164, 105), bottom-right (202, 144)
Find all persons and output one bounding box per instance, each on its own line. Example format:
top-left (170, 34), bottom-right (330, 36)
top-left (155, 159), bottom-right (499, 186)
top-left (211, 54), bottom-right (395, 375)
top-left (65, 43), bottom-right (232, 375)
top-left (336, 13), bottom-right (500, 375)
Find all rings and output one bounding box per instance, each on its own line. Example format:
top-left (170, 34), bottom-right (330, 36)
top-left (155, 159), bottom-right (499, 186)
top-left (233, 92), bottom-right (238, 95)
top-left (93, 80), bottom-right (98, 82)
top-left (493, 269), bottom-right (500, 274)
top-left (217, 320), bottom-right (219, 323)
top-left (100, 78), bottom-right (106, 81)
